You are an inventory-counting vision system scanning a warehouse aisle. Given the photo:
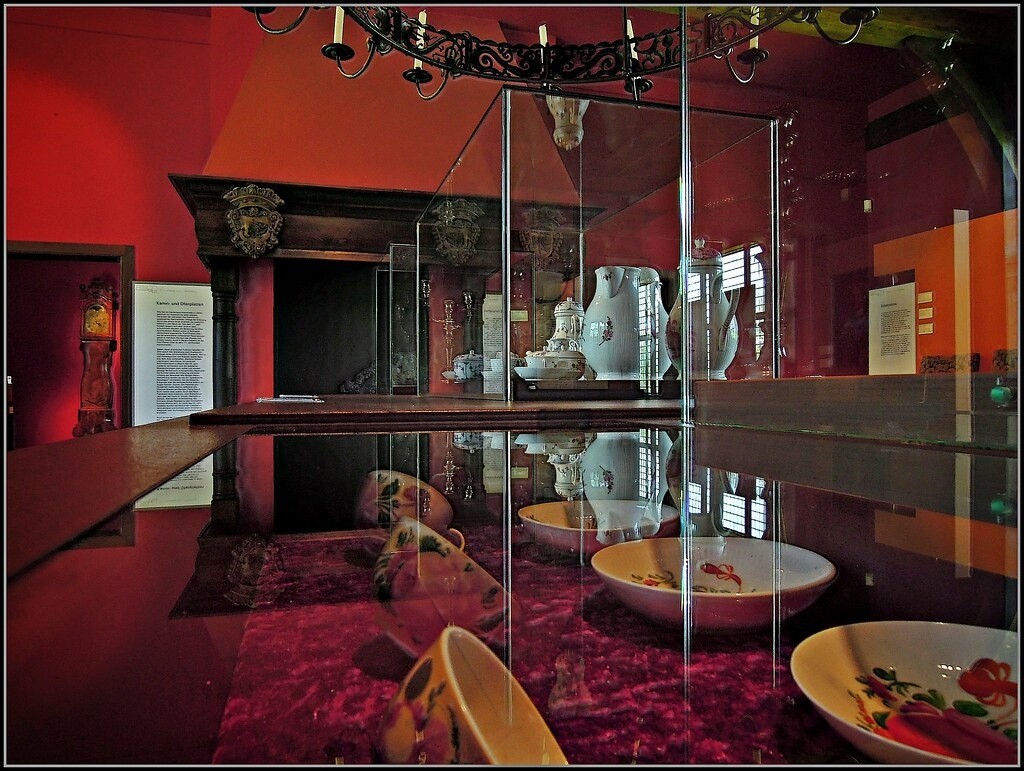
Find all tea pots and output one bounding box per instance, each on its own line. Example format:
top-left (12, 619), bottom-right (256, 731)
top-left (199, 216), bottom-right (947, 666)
top-left (665, 236), bottom-right (740, 380)
top-left (580, 266), bottom-right (657, 380)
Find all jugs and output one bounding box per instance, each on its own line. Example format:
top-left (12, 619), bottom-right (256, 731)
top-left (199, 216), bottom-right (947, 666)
top-left (666, 424), bottom-right (738, 569)
top-left (579, 428), bottom-right (674, 550)
top-left (549, 455), bottom-right (582, 501)
top-left (546, 94), bottom-right (590, 150)
top-left (550, 298), bottom-right (585, 351)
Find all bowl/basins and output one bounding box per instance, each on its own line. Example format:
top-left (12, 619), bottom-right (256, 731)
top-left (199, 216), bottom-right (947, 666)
top-left (558, 432), bottom-right (585, 454)
top-left (559, 350), bottom-right (585, 380)
top-left (451, 348), bottom-right (483, 380)
top-left (453, 430), bottom-right (483, 454)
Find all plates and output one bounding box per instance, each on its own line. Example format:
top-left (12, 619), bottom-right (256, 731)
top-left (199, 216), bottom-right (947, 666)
top-left (790, 621), bottom-right (1019, 766)
top-left (518, 492), bottom-right (679, 555)
top-left (589, 534), bottom-right (840, 635)
top-left (513, 367), bottom-right (571, 380)
top-left (513, 434), bottom-right (568, 444)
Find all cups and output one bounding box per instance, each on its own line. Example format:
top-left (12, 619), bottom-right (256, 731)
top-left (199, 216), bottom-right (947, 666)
top-left (369, 512), bottom-right (519, 661)
top-left (358, 466), bottom-right (465, 555)
top-left (525, 444), bottom-right (553, 455)
top-left (523, 356), bottom-right (559, 367)
top-left (373, 624), bottom-right (571, 770)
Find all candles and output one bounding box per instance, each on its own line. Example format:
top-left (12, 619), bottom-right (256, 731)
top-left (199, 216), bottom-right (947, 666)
top-left (333, 6), bottom-right (345, 44)
top-left (539, 23), bottom-right (547, 72)
top-left (627, 19), bottom-right (642, 80)
top-left (749, 6), bottom-right (759, 49)
top-left (412, 9), bottom-right (427, 69)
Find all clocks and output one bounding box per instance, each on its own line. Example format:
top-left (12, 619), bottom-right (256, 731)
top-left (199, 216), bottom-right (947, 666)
top-left (78, 276), bottom-right (122, 536)
